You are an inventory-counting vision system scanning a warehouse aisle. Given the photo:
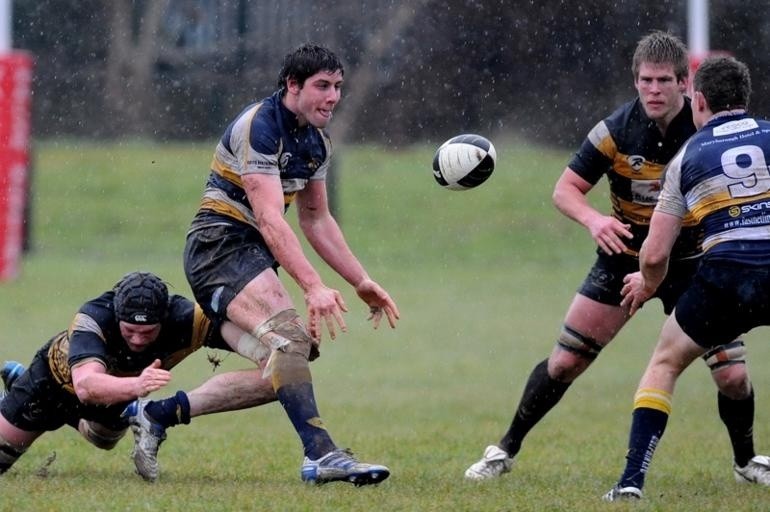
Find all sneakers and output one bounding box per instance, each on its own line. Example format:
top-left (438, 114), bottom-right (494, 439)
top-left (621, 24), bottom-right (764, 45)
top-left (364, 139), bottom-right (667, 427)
top-left (2, 361), bottom-right (26, 399)
top-left (120, 398), bottom-right (167, 481)
top-left (465, 444), bottom-right (515, 481)
top-left (732, 453), bottom-right (770, 487)
top-left (602, 483), bottom-right (641, 502)
top-left (301, 448), bottom-right (391, 488)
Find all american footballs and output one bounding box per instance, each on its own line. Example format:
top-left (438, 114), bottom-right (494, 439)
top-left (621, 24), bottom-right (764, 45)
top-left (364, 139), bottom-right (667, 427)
top-left (432, 134), bottom-right (497, 191)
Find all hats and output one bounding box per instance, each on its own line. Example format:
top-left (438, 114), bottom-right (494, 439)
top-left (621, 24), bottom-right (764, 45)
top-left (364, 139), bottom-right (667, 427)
top-left (113, 272), bottom-right (168, 325)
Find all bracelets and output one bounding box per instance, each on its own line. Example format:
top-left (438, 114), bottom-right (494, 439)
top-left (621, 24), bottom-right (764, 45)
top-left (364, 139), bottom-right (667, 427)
top-left (237, 332), bottom-right (270, 363)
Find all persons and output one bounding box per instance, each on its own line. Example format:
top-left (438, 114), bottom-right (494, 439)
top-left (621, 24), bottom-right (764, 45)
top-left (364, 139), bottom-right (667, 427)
top-left (121, 41), bottom-right (402, 484)
top-left (463, 27), bottom-right (769, 485)
top-left (599, 50), bottom-right (770, 501)
top-left (0, 270), bottom-right (271, 476)
